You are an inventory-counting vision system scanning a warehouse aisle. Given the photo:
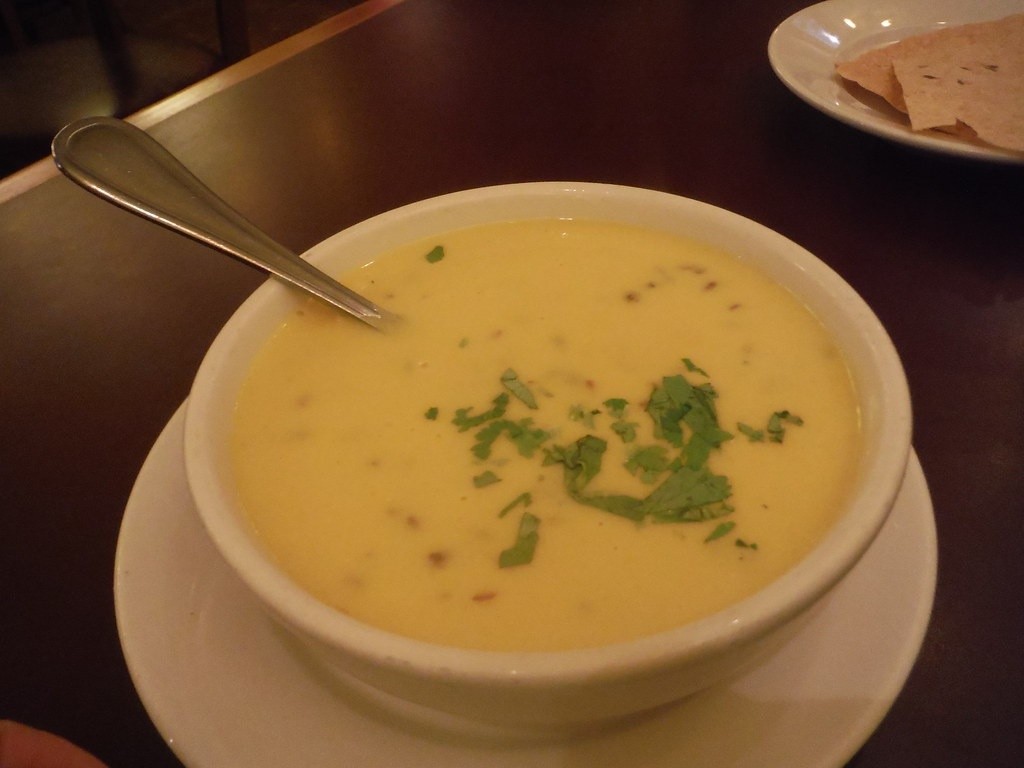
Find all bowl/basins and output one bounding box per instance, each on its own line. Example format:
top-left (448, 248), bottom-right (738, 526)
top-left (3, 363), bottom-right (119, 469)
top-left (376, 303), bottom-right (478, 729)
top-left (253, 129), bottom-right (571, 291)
top-left (184, 183), bottom-right (912, 740)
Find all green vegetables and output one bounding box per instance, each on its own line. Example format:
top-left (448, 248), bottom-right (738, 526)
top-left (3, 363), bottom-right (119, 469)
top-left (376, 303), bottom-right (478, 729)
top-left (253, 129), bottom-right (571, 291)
top-left (421, 246), bottom-right (805, 567)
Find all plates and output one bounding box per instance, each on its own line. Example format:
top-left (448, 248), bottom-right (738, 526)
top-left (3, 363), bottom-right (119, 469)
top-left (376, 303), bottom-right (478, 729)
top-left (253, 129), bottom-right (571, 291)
top-left (768, 0), bottom-right (1024, 164)
top-left (115, 392), bottom-right (937, 767)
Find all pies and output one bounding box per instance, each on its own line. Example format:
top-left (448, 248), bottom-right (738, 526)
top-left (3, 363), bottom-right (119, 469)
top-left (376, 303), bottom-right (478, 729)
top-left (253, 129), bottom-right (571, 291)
top-left (835, 11), bottom-right (1024, 155)
